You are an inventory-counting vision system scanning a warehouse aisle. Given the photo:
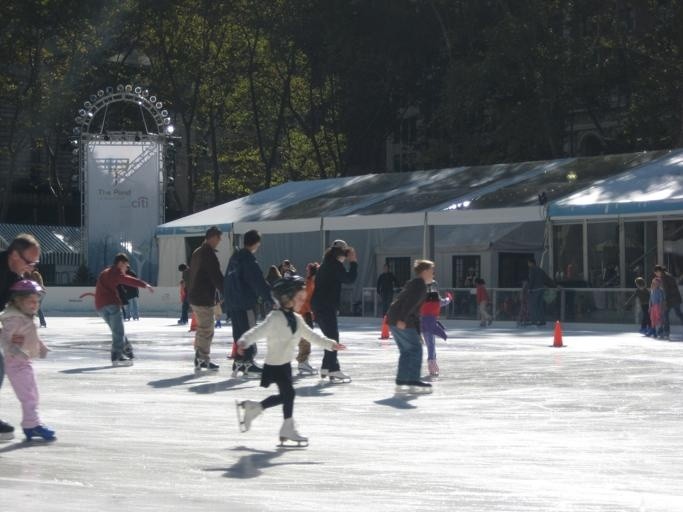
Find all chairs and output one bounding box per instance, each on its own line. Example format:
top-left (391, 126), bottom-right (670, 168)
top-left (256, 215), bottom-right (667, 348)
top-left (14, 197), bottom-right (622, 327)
top-left (298, 362), bottom-right (349, 381)
top-left (24, 424), bottom-right (56, 439)
top-left (112, 354), bottom-right (134, 362)
top-left (195, 357), bottom-right (220, 369)
top-left (232, 360), bottom-right (263, 373)
top-left (427, 358), bottom-right (440, 377)
top-left (393, 380), bottom-right (435, 388)
top-left (0, 418), bottom-right (14, 433)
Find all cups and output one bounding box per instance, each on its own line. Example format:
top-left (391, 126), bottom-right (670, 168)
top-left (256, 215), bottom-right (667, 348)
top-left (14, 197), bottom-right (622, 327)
top-left (20, 254), bottom-right (40, 267)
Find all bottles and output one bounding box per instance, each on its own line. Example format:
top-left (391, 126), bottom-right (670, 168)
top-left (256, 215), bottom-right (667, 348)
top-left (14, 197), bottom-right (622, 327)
top-left (331, 239), bottom-right (351, 251)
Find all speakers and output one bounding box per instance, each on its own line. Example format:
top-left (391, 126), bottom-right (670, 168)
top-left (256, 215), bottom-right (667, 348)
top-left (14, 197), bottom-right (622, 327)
top-left (242, 400), bottom-right (263, 431)
top-left (280, 416), bottom-right (308, 442)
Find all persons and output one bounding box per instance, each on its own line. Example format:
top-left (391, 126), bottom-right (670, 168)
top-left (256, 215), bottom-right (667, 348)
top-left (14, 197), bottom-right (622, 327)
top-left (95, 253), bottom-right (155, 367)
top-left (475, 279), bottom-right (492, 327)
top-left (465, 267), bottom-right (477, 288)
top-left (0, 234), bottom-right (40, 433)
top-left (32, 271), bottom-right (47, 330)
top-left (623, 277), bottom-right (651, 333)
top-left (178, 227), bottom-right (358, 442)
top-left (420, 280), bottom-right (453, 376)
top-left (126, 266), bottom-right (139, 319)
top-left (648, 278), bottom-right (665, 339)
top-left (525, 259), bottom-right (557, 326)
top-left (117, 285), bottom-right (128, 321)
top-left (377, 263), bottom-right (396, 318)
top-left (654, 265), bottom-right (683, 340)
top-left (386, 260), bottom-right (435, 394)
top-left (0, 280), bottom-right (56, 442)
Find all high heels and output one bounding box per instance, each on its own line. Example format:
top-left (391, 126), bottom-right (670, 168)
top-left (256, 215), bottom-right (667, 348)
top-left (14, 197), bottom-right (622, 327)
top-left (549, 319), bottom-right (567, 348)
top-left (188, 310), bottom-right (201, 332)
top-left (377, 313), bottom-right (391, 340)
top-left (228, 339), bottom-right (240, 359)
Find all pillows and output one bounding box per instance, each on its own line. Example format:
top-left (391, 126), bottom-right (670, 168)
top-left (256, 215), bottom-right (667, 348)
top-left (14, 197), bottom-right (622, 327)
top-left (9, 279), bottom-right (47, 295)
top-left (271, 274), bottom-right (306, 295)
